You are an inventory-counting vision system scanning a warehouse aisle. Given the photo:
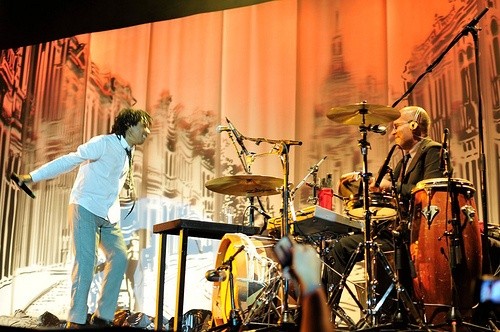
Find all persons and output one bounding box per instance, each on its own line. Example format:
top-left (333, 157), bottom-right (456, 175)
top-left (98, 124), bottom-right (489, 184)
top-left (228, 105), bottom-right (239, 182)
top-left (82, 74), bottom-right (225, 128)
top-left (9, 108), bottom-right (154, 329)
top-left (321, 106), bottom-right (445, 324)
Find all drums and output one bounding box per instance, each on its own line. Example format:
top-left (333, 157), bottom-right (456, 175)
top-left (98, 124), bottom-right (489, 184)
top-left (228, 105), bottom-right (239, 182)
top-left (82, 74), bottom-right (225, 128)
top-left (344, 192), bottom-right (399, 220)
top-left (409, 177), bottom-right (483, 332)
top-left (212, 233), bottom-right (297, 332)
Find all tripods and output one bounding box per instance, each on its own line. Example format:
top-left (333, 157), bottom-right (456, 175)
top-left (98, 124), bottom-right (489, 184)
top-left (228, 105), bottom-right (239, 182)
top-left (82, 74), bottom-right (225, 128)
top-left (210, 111), bottom-right (495, 332)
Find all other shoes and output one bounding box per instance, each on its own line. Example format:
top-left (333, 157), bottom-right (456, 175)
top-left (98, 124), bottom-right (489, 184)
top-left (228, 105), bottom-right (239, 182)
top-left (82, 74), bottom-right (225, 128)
top-left (66, 322), bottom-right (87, 330)
top-left (90, 313), bottom-right (115, 327)
top-left (393, 308), bottom-right (404, 323)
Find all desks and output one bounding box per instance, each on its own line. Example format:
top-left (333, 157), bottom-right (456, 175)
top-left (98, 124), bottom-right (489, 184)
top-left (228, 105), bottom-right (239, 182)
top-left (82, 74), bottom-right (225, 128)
top-left (153, 219), bottom-right (259, 332)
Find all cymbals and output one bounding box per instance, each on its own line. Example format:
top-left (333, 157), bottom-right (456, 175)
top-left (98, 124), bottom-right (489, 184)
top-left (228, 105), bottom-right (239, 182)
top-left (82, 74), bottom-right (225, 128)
top-left (326, 102), bottom-right (402, 127)
top-left (204, 175), bottom-right (295, 197)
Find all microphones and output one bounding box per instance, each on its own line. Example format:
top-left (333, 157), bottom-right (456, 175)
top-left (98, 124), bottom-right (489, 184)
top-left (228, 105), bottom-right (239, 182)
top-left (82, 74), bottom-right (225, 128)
top-left (206, 270), bottom-right (227, 282)
top-left (375, 144), bottom-right (396, 188)
top-left (227, 119), bottom-right (250, 156)
top-left (253, 205), bottom-right (271, 219)
top-left (439, 129), bottom-right (448, 171)
top-left (370, 126), bottom-right (386, 135)
top-left (216, 126), bottom-right (232, 133)
top-left (10, 172), bottom-right (35, 199)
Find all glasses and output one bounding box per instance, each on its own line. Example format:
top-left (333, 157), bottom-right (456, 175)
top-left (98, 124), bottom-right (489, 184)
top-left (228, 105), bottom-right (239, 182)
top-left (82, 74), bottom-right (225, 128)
top-left (393, 120), bottom-right (413, 131)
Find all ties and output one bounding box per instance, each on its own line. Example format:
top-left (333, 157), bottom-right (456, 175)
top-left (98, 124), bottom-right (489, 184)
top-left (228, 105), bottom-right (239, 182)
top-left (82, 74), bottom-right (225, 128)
top-left (398, 153), bottom-right (411, 195)
top-left (117, 135), bottom-right (132, 190)
